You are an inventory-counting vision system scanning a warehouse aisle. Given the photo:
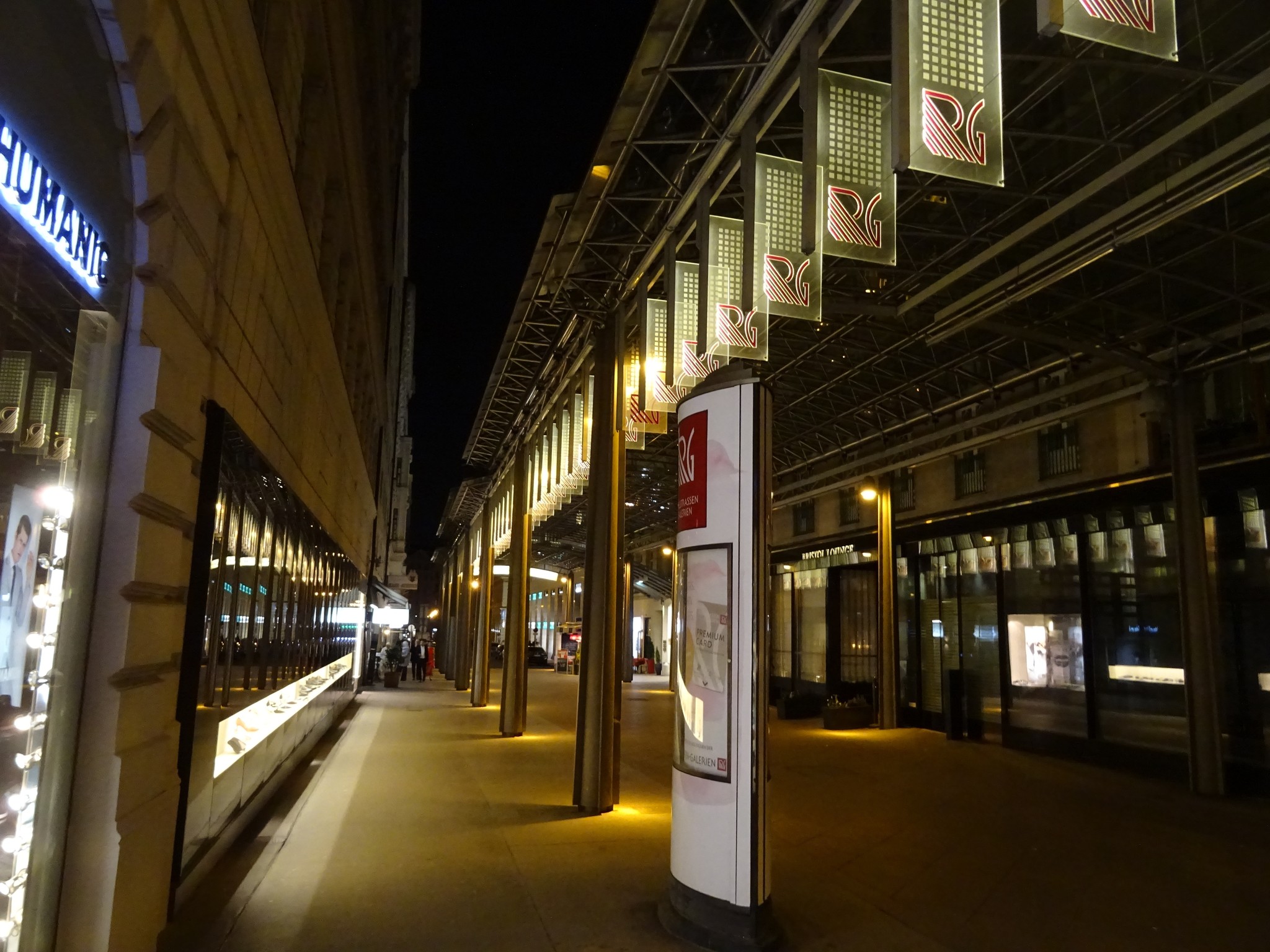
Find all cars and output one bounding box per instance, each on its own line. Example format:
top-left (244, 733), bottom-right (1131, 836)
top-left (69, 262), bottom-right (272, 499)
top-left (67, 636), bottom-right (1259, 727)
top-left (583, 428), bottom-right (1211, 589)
top-left (528, 646), bottom-right (548, 666)
top-left (490, 642), bottom-right (504, 661)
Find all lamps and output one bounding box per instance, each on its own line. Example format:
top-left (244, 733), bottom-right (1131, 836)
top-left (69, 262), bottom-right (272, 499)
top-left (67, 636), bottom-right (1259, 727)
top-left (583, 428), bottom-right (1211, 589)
top-left (3, 486), bottom-right (82, 952)
top-left (860, 475), bottom-right (876, 503)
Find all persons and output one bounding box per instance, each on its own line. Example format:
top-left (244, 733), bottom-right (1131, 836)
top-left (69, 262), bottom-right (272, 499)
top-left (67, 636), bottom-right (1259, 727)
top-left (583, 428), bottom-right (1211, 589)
top-left (399, 641), bottom-right (410, 682)
top-left (0, 513), bottom-right (36, 669)
top-left (379, 642), bottom-right (392, 680)
top-left (411, 639), bottom-right (420, 681)
top-left (426, 640), bottom-right (435, 680)
top-left (417, 638), bottom-right (428, 682)
